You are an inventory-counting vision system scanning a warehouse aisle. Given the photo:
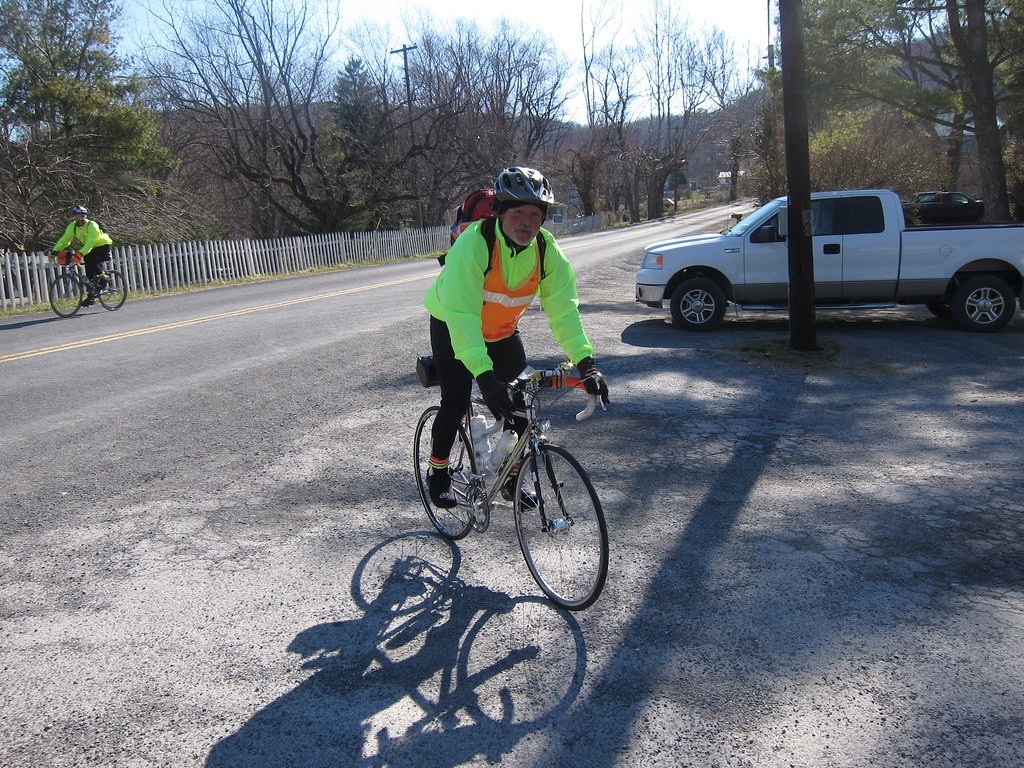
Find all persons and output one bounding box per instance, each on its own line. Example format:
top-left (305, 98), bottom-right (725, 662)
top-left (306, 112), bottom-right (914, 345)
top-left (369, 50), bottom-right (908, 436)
top-left (51, 205), bottom-right (113, 307)
top-left (422, 166), bottom-right (610, 513)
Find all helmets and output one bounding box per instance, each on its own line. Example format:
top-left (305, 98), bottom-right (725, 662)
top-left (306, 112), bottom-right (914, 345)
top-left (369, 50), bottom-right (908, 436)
top-left (70, 205), bottom-right (87, 215)
top-left (493, 167), bottom-right (554, 205)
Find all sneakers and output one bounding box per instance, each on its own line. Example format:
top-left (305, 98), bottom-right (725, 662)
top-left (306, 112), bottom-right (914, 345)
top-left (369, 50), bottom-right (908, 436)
top-left (501, 476), bottom-right (545, 507)
top-left (425, 467), bottom-right (457, 508)
top-left (100, 275), bottom-right (110, 282)
top-left (81, 298), bottom-right (94, 305)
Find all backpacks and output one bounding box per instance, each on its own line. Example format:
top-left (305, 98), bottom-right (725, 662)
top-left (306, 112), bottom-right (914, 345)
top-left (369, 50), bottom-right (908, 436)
top-left (74, 217), bottom-right (108, 238)
top-left (437, 189), bottom-right (547, 280)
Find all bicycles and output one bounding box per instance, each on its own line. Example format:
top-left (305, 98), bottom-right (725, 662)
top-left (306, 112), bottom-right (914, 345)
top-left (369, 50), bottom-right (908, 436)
top-left (43, 245), bottom-right (129, 320)
top-left (412, 361), bottom-right (612, 610)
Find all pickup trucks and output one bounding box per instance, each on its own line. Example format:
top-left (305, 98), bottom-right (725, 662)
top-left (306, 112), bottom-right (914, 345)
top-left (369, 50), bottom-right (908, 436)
top-left (902, 191), bottom-right (985, 224)
top-left (635, 188), bottom-right (1024, 331)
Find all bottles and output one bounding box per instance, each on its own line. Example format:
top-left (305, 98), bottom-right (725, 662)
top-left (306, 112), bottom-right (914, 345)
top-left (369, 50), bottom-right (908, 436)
top-left (82, 275), bottom-right (91, 287)
top-left (470, 411), bottom-right (492, 462)
top-left (91, 279), bottom-right (95, 291)
top-left (490, 429), bottom-right (518, 473)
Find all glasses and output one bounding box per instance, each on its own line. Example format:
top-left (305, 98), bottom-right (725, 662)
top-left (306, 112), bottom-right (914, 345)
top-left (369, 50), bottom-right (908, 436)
top-left (509, 208), bottom-right (545, 222)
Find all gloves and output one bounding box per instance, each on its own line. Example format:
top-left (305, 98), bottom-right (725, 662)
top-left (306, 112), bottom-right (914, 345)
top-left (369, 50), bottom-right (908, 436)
top-left (476, 370), bottom-right (516, 425)
top-left (577, 356), bottom-right (610, 406)
top-left (71, 252), bottom-right (82, 263)
